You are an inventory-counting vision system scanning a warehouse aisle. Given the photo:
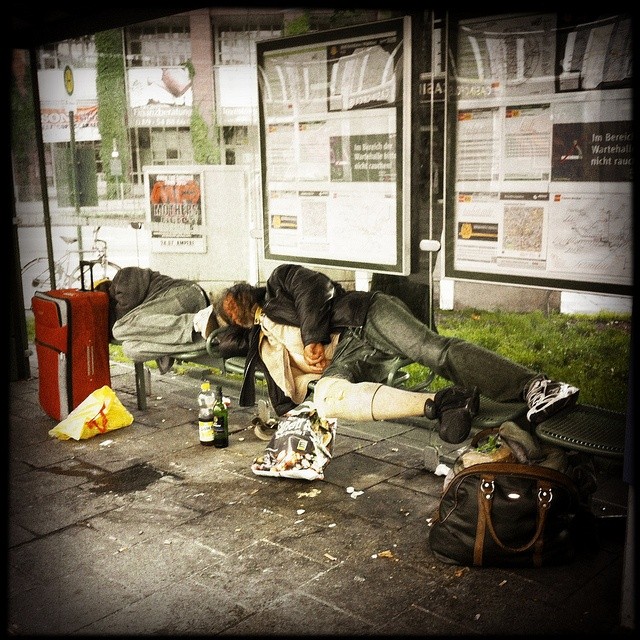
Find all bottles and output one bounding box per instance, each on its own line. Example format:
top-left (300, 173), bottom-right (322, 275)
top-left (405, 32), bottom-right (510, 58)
top-left (198, 383), bottom-right (215, 446)
top-left (212, 384), bottom-right (228, 448)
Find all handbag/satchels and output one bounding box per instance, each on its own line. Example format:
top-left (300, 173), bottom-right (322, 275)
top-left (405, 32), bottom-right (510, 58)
top-left (430, 463), bottom-right (581, 569)
top-left (48, 385), bottom-right (134, 440)
top-left (251, 399), bottom-right (338, 480)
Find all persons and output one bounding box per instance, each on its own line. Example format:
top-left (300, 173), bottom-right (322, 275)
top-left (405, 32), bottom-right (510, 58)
top-left (216, 264), bottom-right (580, 445)
top-left (95, 267), bottom-right (220, 362)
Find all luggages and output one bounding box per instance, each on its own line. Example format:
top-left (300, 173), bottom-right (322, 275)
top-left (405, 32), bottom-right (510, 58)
top-left (31, 261), bottom-right (111, 422)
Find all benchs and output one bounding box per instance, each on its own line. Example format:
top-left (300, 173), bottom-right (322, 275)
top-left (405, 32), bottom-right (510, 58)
top-left (108, 325), bottom-right (205, 413)
top-left (206, 325), bottom-right (438, 391)
top-left (382, 377), bottom-right (639, 459)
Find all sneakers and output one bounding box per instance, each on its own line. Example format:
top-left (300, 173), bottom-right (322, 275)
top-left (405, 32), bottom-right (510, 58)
top-left (193, 304), bottom-right (218, 339)
top-left (525, 377), bottom-right (579, 424)
top-left (423, 386), bottom-right (482, 444)
